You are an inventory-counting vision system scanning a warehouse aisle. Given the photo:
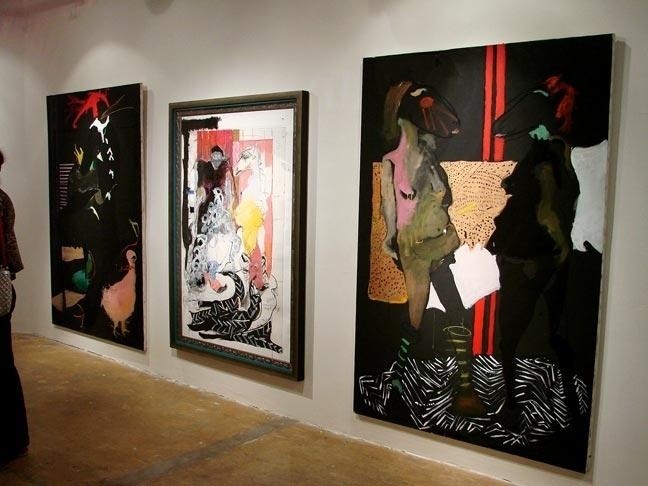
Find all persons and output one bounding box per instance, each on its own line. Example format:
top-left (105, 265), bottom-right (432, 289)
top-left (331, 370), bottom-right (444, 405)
top-left (0, 152), bottom-right (30, 470)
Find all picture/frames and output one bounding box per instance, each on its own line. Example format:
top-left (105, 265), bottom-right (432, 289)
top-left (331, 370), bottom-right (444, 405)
top-left (166, 89), bottom-right (310, 382)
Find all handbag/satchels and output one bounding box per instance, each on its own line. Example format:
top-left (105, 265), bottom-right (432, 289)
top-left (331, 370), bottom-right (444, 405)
top-left (0, 265), bottom-right (12, 317)
